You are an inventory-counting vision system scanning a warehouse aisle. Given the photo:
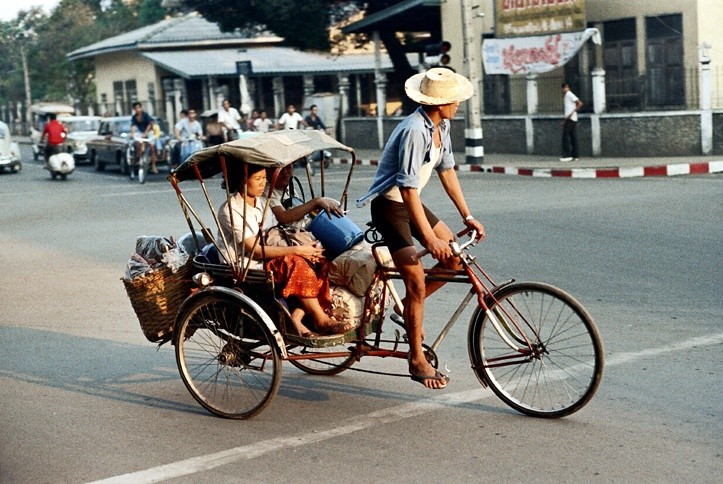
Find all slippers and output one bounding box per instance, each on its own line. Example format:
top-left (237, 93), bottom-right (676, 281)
top-left (411, 366), bottom-right (450, 388)
top-left (390, 314), bottom-right (405, 329)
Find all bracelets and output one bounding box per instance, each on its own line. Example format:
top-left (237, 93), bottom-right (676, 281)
top-left (463, 215), bottom-right (474, 224)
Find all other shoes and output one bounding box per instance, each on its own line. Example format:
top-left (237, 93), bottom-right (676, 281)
top-left (302, 331), bottom-right (322, 337)
top-left (559, 156), bottom-right (583, 162)
top-left (314, 318), bottom-right (353, 334)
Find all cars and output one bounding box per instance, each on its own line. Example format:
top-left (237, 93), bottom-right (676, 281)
top-left (85, 116), bottom-right (171, 177)
top-left (59, 116), bottom-right (102, 161)
top-left (0, 119), bottom-right (23, 172)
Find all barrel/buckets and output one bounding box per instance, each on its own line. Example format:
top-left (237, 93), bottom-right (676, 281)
top-left (304, 207), bottom-right (364, 261)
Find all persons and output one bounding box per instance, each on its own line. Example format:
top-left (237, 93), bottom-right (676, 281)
top-left (170, 108), bottom-right (231, 170)
top-left (304, 104), bottom-right (325, 131)
top-left (241, 111), bottom-right (274, 132)
top-left (272, 103), bottom-right (310, 130)
top-left (39, 114), bottom-right (67, 169)
top-left (218, 160), bottom-right (353, 339)
top-left (559, 82), bottom-right (584, 161)
top-left (130, 102), bottom-right (158, 174)
top-left (217, 99), bottom-right (243, 135)
top-left (357, 68), bottom-right (485, 390)
top-left (261, 163), bottom-right (344, 227)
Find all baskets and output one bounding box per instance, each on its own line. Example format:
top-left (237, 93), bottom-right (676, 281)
top-left (120, 257), bottom-right (194, 343)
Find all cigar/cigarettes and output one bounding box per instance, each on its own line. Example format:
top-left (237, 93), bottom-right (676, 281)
top-left (343, 211), bottom-right (349, 213)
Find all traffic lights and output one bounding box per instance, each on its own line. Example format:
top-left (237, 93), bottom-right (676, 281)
top-left (418, 41), bottom-right (456, 73)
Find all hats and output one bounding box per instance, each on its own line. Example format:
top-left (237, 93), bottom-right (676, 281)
top-left (404, 68), bottom-right (474, 105)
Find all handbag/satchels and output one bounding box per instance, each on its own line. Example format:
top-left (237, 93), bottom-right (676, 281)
top-left (262, 223), bottom-right (317, 246)
top-left (280, 176), bottom-right (306, 211)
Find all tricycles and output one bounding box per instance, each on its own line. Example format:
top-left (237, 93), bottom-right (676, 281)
top-left (28, 102), bottom-right (75, 161)
top-left (166, 128), bottom-right (605, 421)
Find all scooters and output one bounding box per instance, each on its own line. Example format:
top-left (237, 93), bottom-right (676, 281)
top-left (39, 141), bottom-right (75, 180)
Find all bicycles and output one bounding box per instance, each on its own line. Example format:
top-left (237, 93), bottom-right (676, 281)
top-left (121, 133), bottom-right (150, 185)
